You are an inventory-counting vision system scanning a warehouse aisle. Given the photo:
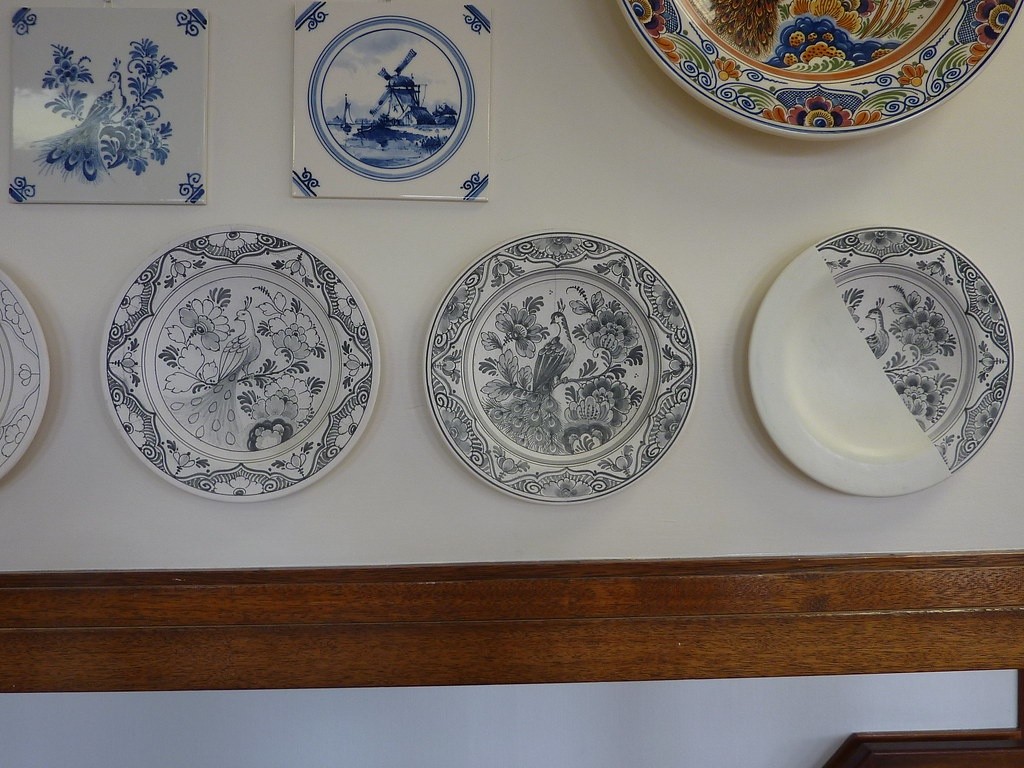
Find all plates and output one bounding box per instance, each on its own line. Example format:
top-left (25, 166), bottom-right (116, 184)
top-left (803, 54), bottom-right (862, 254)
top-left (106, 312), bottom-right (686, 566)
top-left (615, 0), bottom-right (1024, 142)
top-left (747, 227), bottom-right (1015, 498)
top-left (98, 224), bottom-right (375, 505)
top-left (0, 270), bottom-right (50, 481)
top-left (422, 229), bottom-right (698, 505)
